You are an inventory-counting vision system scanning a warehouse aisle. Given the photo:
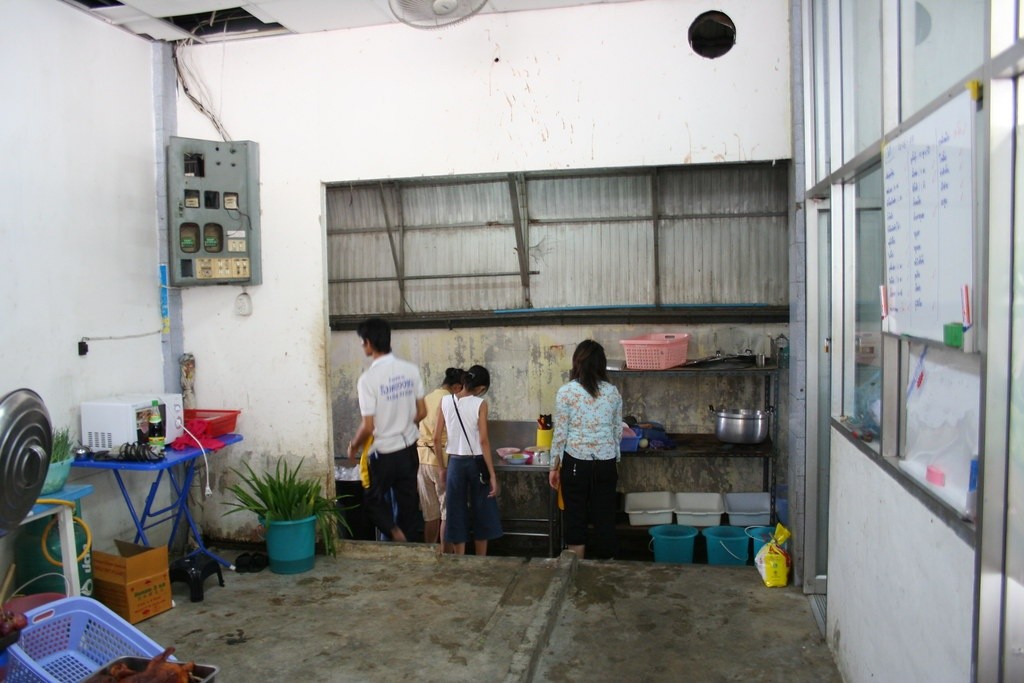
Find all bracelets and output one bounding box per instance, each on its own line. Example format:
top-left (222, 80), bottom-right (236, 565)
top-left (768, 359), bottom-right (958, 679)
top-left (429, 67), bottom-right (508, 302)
top-left (551, 468), bottom-right (558, 471)
top-left (349, 441), bottom-right (356, 450)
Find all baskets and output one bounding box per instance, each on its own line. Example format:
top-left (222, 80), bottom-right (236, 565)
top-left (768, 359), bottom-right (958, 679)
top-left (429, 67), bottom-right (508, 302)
top-left (619, 332), bottom-right (690, 370)
top-left (5, 596), bottom-right (180, 683)
top-left (184, 408), bottom-right (241, 439)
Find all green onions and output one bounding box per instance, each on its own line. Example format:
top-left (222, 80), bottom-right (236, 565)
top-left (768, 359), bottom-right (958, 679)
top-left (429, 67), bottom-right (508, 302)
top-left (50, 427), bottom-right (74, 464)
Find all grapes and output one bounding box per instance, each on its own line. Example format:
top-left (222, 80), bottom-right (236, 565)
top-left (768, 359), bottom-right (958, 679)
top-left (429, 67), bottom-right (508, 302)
top-left (0, 608), bottom-right (29, 635)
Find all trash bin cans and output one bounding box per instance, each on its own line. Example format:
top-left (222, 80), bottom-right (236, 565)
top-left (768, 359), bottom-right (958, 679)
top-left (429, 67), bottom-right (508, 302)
top-left (337, 480), bottom-right (377, 540)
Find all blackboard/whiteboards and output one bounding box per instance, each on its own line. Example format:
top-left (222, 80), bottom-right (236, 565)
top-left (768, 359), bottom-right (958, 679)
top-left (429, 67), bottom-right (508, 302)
top-left (880, 77), bottom-right (978, 354)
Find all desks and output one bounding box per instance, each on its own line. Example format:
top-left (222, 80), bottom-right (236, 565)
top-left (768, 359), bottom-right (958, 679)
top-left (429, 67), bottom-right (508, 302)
top-left (0, 483), bottom-right (93, 598)
top-left (491, 453), bottom-right (560, 559)
top-left (70, 433), bottom-right (244, 570)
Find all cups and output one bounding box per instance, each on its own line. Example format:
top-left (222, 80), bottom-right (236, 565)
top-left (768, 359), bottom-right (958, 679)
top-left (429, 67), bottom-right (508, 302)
top-left (756, 354), bottom-right (765, 368)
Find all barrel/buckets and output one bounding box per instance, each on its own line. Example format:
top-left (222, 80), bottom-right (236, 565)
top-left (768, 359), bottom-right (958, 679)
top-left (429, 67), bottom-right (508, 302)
top-left (745, 526), bottom-right (791, 566)
top-left (335, 480), bottom-right (377, 541)
top-left (537, 428), bottom-right (554, 449)
top-left (702, 526), bottom-right (749, 566)
top-left (649, 525), bottom-right (698, 565)
top-left (258, 515), bottom-right (320, 574)
top-left (4, 573), bottom-right (70, 662)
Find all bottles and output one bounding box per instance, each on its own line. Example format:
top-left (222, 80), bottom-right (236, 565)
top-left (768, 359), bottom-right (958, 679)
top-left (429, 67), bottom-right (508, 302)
top-left (522, 447), bottom-right (536, 464)
top-left (863, 383), bottom-right (877, 427)
top-left (778, 348), bottom-right (784, 368)
top-left (148, 400), bottom-right (165, 453)
top-left (532, 451), bottom-right (550, 465)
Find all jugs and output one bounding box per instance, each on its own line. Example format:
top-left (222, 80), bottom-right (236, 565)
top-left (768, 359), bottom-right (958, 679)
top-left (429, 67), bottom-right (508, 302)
top-left (767, 332), bottom-right (790, 364)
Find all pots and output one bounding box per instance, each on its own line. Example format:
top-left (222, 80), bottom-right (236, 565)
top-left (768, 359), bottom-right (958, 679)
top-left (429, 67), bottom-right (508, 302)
top-left (709, 405), bottom-right (774, 444)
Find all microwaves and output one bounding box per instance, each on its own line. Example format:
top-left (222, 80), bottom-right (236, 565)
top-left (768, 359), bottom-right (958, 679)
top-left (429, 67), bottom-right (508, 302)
top-left (80, 392), bottom-right (184, 450)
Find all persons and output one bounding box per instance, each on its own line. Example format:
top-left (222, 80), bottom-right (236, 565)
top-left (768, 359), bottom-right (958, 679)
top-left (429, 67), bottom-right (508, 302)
top-left (549, 340), bottom-right (624, 560)
top-left (416, 368), bottom-right (465, 543)
top-left (434, 365), bottom-right (504, 556)
top-left (347, 317), bottom-right (428, 543)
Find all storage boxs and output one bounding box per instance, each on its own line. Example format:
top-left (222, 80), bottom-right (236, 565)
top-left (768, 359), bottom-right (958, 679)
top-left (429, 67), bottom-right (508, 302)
top-left (620, 333), bottom-right (690, 370)
top-left (6, 596), bottom-right (177, 683)
top-left (92, 539), bottom-right (172, 624)
top-left (80, 656), bottom-right (220, 683)
top-left (625, 492), bottom-right (778, 526)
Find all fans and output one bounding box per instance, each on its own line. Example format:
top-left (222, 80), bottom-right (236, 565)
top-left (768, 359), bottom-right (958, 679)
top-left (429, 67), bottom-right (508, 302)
top-left (388, 0), bottom-right (487, 29)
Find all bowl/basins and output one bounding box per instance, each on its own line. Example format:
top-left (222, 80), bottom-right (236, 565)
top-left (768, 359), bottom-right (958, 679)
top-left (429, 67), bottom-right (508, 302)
top-left (504, 454), bottom-right (529, 465)
top-left (496, 448), bottom-right (521, 457)
top-left (40, 457), bottom-right (74, 495)
